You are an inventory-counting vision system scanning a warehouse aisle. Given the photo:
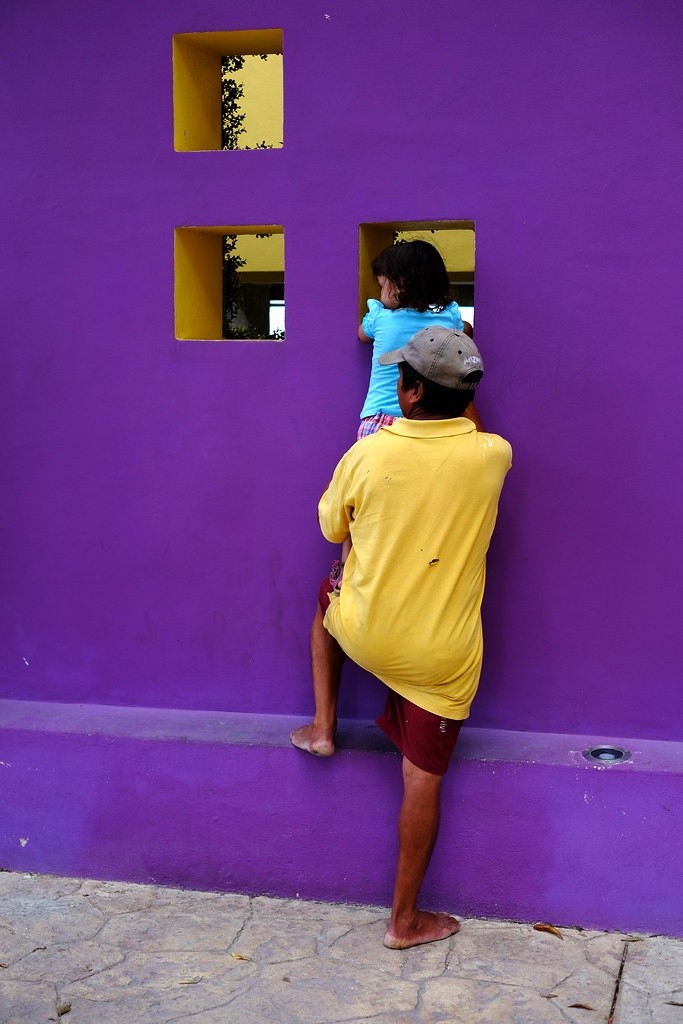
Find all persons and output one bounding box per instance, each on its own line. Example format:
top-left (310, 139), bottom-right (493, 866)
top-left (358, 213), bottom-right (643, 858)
top-left (289, 326), bottom-right (512, 949)
top-left (328, 240), bottom-right (485, 595)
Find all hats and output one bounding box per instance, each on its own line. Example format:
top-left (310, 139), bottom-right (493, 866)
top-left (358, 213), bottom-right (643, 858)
top-left (378, 326), bottom-right (484, 389)
